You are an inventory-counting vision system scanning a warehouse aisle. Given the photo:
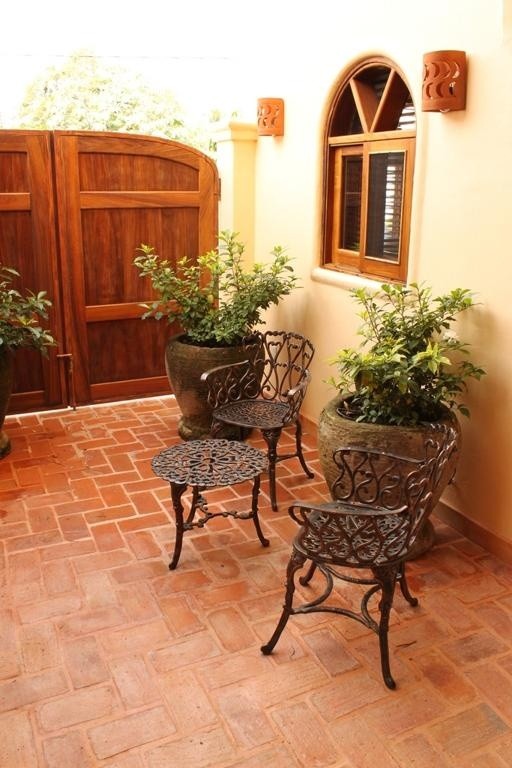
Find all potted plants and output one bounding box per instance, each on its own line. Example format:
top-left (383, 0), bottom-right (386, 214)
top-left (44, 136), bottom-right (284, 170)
top-left (0, 266), bottom-right (57, 457)
top-left (132, 228), bottom-right (304, 444)
top-left (316, 281), bottom-right (486, 561)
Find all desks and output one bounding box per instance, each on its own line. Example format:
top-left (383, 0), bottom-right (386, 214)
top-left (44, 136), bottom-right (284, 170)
top-left (151, 439), bottom-right (269, 570)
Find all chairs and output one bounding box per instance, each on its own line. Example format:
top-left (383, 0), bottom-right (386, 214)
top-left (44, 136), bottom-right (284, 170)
top-left (200, 330), bottom-right (314, 512)
top-left (260, 422), bottom-right (458, 689)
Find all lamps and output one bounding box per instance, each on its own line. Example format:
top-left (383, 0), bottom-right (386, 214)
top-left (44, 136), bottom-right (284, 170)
top-left (257, 98), bottom-right (284, 137)
top-left (421, 50), bottom-right (467, 113)
